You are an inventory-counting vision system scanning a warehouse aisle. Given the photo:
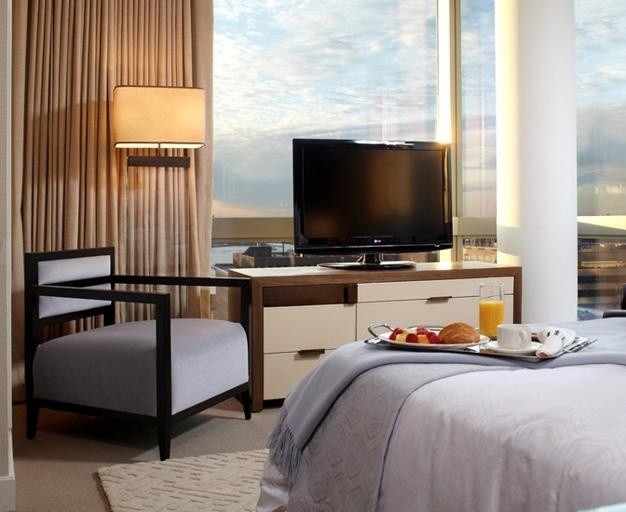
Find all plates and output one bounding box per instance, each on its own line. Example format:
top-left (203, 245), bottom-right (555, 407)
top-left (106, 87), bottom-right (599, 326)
top-left (488, 340), bottom-right (541, 355)
top-left (379, 328), bottom-right (491, 348)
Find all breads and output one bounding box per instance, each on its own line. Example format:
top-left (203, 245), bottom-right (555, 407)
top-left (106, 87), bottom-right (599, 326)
top-left (439, 322), bottom-right (480, 343)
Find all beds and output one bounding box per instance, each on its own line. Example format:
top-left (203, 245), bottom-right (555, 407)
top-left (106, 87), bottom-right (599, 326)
top-left (335, 318), bottom-right (626, 512)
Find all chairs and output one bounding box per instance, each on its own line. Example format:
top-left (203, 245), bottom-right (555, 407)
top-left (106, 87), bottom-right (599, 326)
top-left (23, 247), bottom-right (254, 461)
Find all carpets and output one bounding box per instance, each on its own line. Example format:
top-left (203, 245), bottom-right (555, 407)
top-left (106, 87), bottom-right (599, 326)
top-left (96, 449), bottom-right (269, 512)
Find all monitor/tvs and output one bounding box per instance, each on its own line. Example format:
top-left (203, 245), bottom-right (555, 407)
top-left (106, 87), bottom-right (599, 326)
top-left (293, 138), bottom-right (453, 270)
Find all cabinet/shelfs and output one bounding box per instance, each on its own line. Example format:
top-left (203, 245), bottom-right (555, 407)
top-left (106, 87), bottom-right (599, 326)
top-left (230, 263), bottom-right (523, 413)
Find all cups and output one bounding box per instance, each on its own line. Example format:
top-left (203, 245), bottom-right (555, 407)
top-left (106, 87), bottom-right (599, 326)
top-left (477, 283), bottom-right (505, 339)
top-left (497, 323), bottom-right (531, 349)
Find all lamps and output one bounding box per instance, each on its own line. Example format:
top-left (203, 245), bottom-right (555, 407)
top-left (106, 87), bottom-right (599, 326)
top-left (112, 84), bottom-right (206, 169)
